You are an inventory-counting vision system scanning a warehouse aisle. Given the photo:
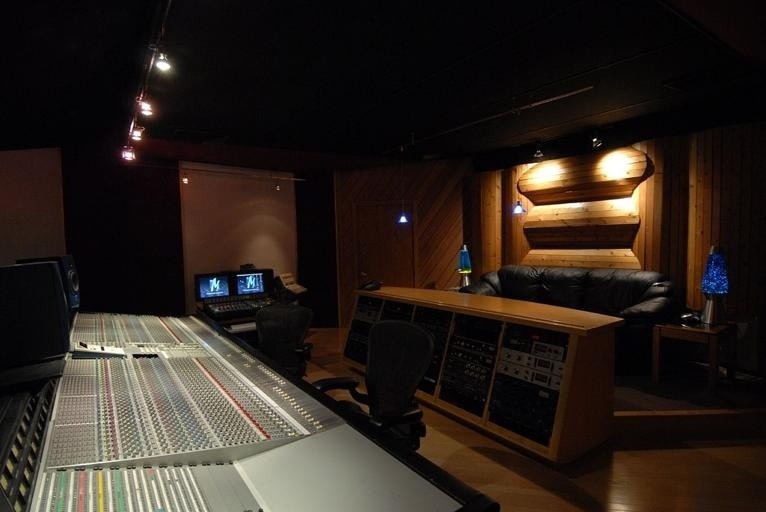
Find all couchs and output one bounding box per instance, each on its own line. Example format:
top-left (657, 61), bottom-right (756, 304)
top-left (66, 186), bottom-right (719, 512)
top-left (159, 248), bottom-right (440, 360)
top-left (458, 263), bottom-right (673, 387)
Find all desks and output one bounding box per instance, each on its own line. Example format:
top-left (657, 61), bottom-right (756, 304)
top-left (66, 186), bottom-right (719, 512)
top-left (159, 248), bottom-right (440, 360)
top-left (339, 286), bottom-right (624, 463)
top-left (651, 320), bottom-right (738, 393)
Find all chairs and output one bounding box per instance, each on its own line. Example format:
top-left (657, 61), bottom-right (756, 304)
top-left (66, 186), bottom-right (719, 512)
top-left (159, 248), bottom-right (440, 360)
top-left (255, 306), bottom-right (313, 385)
top-left (312, 319), bottom-right (436, 450)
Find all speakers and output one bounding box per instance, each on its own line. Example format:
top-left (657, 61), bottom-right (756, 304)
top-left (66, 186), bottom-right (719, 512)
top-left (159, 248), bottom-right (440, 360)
top-left (16, 255), bottom-right (80, 314)
top-left (0, 261), bottom-right (71, 371)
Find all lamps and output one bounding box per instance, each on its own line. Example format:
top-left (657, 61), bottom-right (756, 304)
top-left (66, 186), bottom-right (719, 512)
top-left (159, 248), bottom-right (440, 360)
top-left (700, 245), bottom-right (730, 324)
top-left (397, 197), bottom-right (409, 225)
top-left (458, 244), bottom-right (473, 287)
top-left (533, 143), bottom-right (544, 158)
top-left (512, 192), bottom-right (526, 215)
top-left (592, 137), bottom-right (607, 151)
top-left (120, 43), bottom-right (171, 161)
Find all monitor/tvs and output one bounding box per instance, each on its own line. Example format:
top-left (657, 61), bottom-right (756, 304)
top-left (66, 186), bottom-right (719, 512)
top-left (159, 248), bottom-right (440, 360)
top-left (235, 272), bottom-right (265, 296)
top-left (194, 272), bottom-right (230, 302)
